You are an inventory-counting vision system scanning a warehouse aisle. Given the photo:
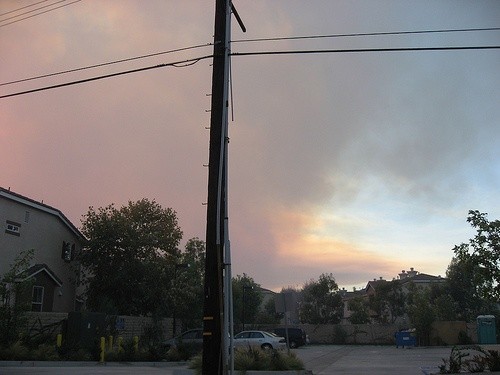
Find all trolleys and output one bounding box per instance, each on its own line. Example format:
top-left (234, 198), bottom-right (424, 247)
top-left (392, 327), bottom-right (416, 350)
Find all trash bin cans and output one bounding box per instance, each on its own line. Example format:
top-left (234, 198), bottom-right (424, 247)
top-left (477, 315), bottom-right (496, 344)
top-left (393, 329), bottom-right (417, 348)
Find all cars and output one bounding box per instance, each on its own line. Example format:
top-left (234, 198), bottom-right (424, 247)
top-left (228, 329), bottom-right (286, 354)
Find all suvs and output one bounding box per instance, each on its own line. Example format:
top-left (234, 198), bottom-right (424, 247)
top-left (269, 327), bottom-right (305, 350)
top-left (164, 327), bottom-right (205, 357)
